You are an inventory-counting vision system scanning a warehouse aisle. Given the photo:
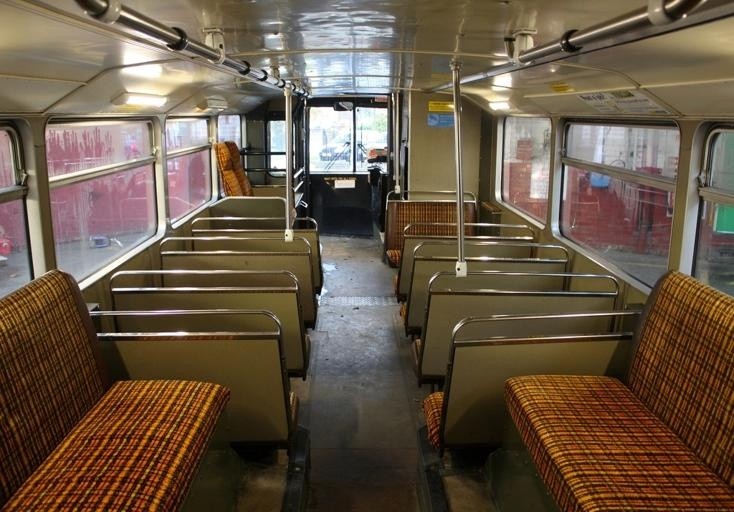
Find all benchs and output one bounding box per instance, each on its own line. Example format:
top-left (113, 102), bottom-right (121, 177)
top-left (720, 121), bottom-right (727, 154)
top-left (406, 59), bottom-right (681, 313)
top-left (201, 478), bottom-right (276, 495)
top-left (1, 270), bottom-right (228, 512)
top-left (505, 270), bottom-right (734, 511)
top-left (209, 195), bottom-right (289, 230)
top-left (394, 222), bottom-right (535, 318)
top-left (411, 271), bottom-right (620, 394)
top-left (400, 240), bottom-right (570, 341)
top-left (159, 237), bottom-right (318, 332)
top-left (85, 307), bottom-right (300, 464)
top-left (190, 217), bottom-right (317, 295)
top-left (423, 310), bottom-right (642, 451)
top-left (384, 190), bottom-right (478, 269)
top-left (108, 270), bottom-right (311, 381)
top-left (216, 142), bottom-right (295, 226)
